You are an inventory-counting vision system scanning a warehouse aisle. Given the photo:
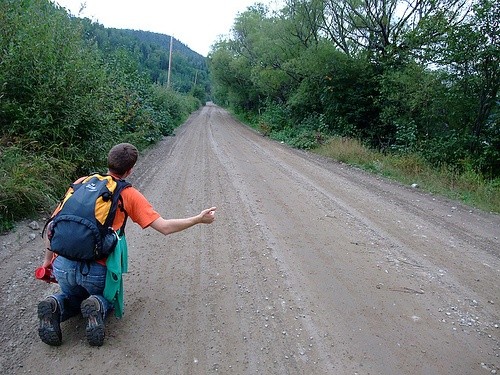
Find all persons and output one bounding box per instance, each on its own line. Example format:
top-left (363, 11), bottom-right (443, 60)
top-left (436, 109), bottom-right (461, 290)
top-left (37, 143), bottom-right (216, 346)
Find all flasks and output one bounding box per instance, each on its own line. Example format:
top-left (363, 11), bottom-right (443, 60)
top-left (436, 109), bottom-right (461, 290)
top-left (36, 268), bottom-right (61, 283)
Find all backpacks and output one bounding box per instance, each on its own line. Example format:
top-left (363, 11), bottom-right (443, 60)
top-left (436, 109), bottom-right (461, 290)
top-left (47, 171), bottom-right (131, 265)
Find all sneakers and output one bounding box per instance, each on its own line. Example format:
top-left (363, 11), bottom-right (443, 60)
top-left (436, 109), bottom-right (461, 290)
top-left (80, 296), bottom-right (106, 346)
top-left (37, 297), bottom-right (62, 346)
